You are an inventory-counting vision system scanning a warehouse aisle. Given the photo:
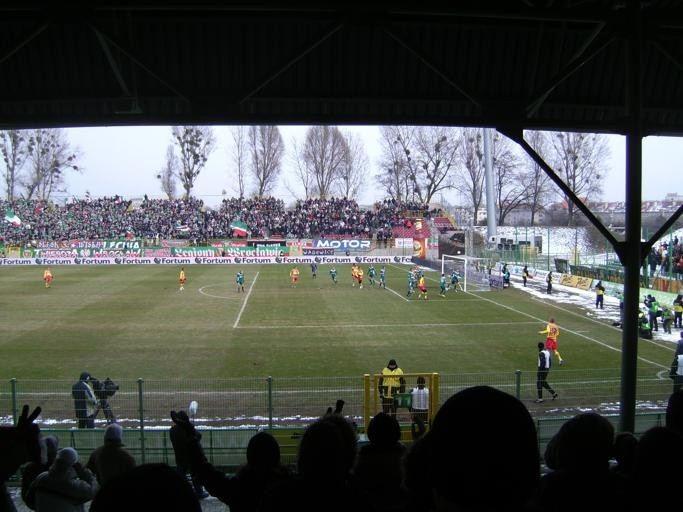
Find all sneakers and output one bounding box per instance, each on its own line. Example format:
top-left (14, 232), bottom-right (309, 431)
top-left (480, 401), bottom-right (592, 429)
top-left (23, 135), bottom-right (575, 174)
top-left (552, 392), bottom-right (558, 401)
top-left (559, 360), bottom-right (564, 366)
top-left (533, 398), bottom-right (544, 404)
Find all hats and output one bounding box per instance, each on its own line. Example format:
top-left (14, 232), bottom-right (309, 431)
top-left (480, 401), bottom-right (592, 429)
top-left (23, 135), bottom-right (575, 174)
top-left (56, 446), bottom-right (79, 464)
top-left (39, 435), bottom-right (59, 450)
top-left (103, 423), bottom-right (123, 442)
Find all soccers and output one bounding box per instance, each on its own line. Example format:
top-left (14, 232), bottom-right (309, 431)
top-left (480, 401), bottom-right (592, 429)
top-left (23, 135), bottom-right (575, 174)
top-left (457, 251), bottom-right (460, 254)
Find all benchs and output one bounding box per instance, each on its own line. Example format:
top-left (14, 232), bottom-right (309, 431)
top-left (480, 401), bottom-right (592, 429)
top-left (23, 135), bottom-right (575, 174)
top-left (590, 254), bottom-right (623, 271)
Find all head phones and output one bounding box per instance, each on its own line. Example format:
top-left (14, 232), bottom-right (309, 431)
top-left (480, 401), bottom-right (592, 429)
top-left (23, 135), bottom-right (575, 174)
top-left (86, 373), bottom-right (90, 380)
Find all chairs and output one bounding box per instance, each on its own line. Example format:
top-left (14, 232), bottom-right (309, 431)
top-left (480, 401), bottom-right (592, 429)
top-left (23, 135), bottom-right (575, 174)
top-left (220, 195), bottom-right (456, 247)
top-left (631, 306), bottom-right (683, 345)
